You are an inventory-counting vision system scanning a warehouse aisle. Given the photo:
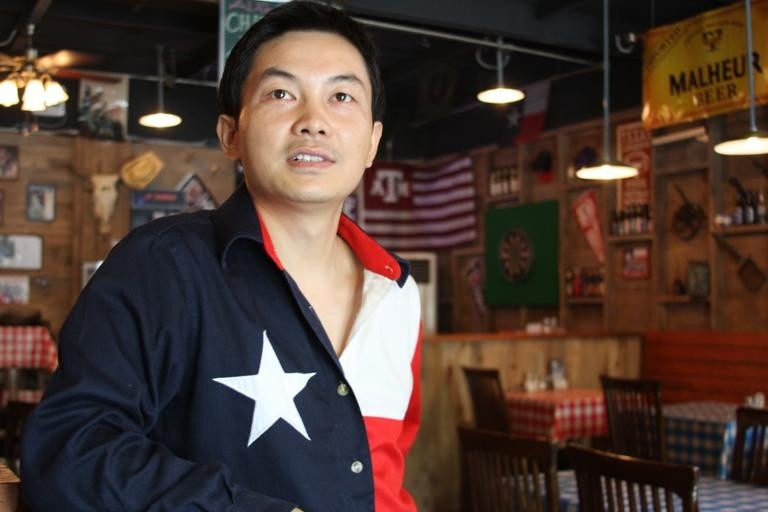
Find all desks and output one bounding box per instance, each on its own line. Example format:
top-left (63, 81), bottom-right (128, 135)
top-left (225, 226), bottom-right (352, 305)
top-left (498, 469), bottom-right (767, 512)
top-left (495, 388), bottom-right (649, 450)
top-left (606, 400), bottom-right (768, 481)
top-left (0, 326), bottom-right (60, 403)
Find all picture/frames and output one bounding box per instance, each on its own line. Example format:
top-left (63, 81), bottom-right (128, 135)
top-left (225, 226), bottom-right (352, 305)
top-left (0, 233), bottom-right (43, 271)
top-left (0, 143), bottom-right (20, 181)
top-left (80, 260), bottom-right (100, 290)
top-left (25, 182), bottom-right (56, 222)
top-left (1, 273), bottom-right (31, 305)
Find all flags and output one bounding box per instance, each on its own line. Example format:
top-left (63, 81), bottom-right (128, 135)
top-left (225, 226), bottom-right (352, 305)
top-left (357, 154), bottom-right (480, 247)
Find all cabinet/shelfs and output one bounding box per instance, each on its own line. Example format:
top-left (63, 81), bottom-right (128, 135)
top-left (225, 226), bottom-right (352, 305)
top-left (450, 103), bottom-right (768, 335)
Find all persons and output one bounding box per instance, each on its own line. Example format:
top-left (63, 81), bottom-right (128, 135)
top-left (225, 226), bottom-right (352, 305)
top-left (18, 1), bottom-right (421, 512)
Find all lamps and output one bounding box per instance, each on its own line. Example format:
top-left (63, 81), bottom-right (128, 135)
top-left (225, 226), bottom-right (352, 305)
top-left (575, 0), bottom-right (639, 180)
top-left (712, 0), bottom-right (766, 155)
top-left (477, 49), bottom-right (524, 104)
top-left (137, 43), bottom-right (182, 129)
top-left (0, 70), bottom-right (69, 136)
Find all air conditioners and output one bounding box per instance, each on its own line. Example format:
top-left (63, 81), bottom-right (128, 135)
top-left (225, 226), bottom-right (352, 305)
top-left (398, 251), bottom-right (438, 333)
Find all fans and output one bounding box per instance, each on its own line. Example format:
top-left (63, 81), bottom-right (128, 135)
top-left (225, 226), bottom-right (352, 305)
top-left (1, 21), bottom-right (122, 87)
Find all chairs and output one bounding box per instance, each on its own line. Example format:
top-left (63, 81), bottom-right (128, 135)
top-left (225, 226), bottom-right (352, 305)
top-left (598, 372), bottom-right (667, 460)
top-left (455, 425), bottom-right (561, 512)
top-left (725, 405), bottom-right (767, 491)
top-left (457, 364), bottom-right (511, 435)
top-left (568, 441), bottom-right (699, 512)
